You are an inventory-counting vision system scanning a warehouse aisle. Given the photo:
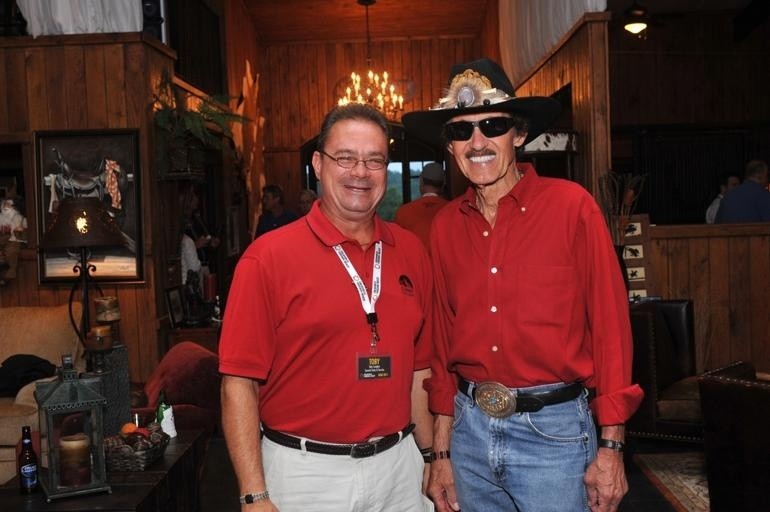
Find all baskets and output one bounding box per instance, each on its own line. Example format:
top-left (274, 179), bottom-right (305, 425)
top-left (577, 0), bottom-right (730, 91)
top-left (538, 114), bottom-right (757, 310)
top-left (105, 433), bottom-right (170, 472)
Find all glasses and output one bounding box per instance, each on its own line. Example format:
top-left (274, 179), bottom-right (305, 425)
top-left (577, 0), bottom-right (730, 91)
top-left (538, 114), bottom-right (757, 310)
top-left (318, 149), bottom-right (388, 171)
top-left (443, 117), bottom-right (513, 141)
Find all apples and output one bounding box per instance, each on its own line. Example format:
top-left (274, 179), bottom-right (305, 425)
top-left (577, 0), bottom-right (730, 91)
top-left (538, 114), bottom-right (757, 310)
top-left (134, 428), bottom-right (148, 437)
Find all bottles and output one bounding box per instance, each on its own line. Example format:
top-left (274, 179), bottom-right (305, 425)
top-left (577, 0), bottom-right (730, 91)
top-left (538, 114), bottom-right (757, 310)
top-left (213, 296), bottom-right (222, 318)
top-left (156, 388), bottom-right (170, 424)
top-left (17, 424), bottom-right (38, 493)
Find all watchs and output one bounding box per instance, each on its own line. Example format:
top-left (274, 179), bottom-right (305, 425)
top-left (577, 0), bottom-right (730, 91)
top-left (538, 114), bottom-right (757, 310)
top-left (240, 491), bottom-right (269, 504)
top-left (599, 439), bottom-right (625, 452)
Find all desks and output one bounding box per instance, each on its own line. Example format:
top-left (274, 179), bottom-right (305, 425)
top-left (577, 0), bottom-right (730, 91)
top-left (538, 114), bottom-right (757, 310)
top-left (0, 419), bottom-right (208, 512)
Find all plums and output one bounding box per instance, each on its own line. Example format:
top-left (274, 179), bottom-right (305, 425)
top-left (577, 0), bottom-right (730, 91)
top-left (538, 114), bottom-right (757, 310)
top-left (125, 432), bottom-right (146, 446)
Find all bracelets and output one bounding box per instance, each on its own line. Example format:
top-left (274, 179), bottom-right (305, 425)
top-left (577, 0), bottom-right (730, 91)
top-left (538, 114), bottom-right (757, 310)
top-left (423, 456), bottom-right (433, 463)
top-left (420, 447), bottom-right (433, 454)
top-left (434, 450), bottom-right (450, 460)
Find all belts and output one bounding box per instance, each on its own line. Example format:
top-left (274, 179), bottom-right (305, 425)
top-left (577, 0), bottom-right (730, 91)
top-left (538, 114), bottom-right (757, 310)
top-left (261, 425), bottom-right (416, 458)
top-left (457, 381), bottom-right (582, 417)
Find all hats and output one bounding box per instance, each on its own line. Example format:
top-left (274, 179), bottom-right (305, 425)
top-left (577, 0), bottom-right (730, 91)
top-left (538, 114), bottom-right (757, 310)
top-left (402, 60), bottom-right (562, 147)
top-left (422, 164), bottom-right (445, 180)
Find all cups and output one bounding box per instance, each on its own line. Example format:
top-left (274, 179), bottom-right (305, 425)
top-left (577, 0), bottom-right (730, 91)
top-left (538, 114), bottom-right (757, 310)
top-left (202, 273), bottom-right (217, 302)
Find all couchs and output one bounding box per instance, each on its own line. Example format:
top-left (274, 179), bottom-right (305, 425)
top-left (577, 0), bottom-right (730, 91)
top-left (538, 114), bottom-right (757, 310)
top-left (0, 301), bottom-right (83, 486)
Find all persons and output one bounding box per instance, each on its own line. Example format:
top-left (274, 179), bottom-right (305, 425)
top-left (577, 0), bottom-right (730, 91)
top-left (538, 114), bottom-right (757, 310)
top-left (218, 103), bottom-right (434, 512)
top-left (181, 190), bottom-right (220, 284)
top-left (402, 57), bottom-right (644, 512)
top-left (705, 158), bottom-right (769, 224)
top-left (392, 162), bottom-right (450, 257)
top-left (254, 183), bottom-right (300, 242)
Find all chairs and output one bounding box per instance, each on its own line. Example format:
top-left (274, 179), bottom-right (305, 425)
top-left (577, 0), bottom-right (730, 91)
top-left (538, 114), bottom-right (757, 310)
top-left (696, 360), bottom-right (770, 512)
top-left (16, 341), bottom-right (222, 477)
top-left (626, 299), bottom-right (705, 453)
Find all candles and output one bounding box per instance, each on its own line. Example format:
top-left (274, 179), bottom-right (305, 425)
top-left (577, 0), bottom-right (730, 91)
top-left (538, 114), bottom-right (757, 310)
top-left (59, 432), bottom-right (92, 485)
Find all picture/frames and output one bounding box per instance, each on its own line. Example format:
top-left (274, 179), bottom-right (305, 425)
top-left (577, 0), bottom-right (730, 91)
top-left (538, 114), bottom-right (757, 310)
top-left (32, 127), bottom-right (147, 287)
top-left (163, 286), bottom-right (185, 330)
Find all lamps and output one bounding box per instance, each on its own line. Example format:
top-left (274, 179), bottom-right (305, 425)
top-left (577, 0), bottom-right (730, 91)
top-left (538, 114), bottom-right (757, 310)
top-left (622, 3), bottom-right (649, 34)
top-left (337, 0), bottom-right (404, 121)
